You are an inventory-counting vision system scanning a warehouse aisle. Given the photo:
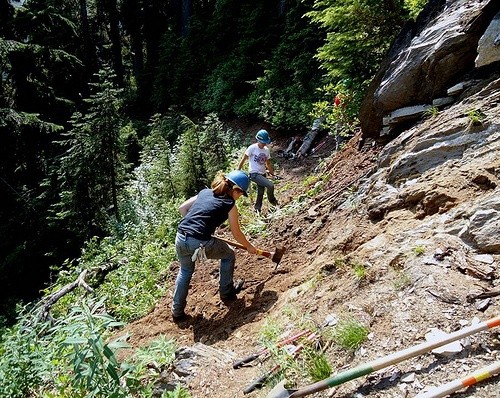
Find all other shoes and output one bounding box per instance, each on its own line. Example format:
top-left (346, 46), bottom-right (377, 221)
top-left (254, 208), bottom-right (261, 214)
top-left (172, 315), bottom-right (183, 321)
top-left (221, 280), bottom-right (245, 299)
top-left (268, 200), bottom-right (278, 206)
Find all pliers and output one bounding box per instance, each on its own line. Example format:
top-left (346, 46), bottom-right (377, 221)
top-left (233, 312), bottom-right (340, 393)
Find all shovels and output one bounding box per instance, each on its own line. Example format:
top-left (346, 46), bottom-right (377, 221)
top-left (264, 316), bottom-right (500, 398)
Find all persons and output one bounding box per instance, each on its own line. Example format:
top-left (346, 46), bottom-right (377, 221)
top-left (236, 130), bottom-right (281, 213)
top-left (171, 170), bottom-right (257, 323)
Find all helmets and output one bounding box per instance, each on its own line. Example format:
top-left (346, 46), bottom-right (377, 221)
top-left (225, 170), bottom-right (249, 197)
top-left (256, 130), bottom-right (270, 143)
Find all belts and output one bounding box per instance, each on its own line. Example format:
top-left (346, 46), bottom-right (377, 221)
top-left (178, 229), bottom-right (192, 237)
